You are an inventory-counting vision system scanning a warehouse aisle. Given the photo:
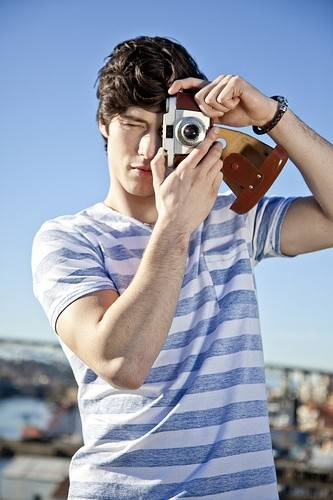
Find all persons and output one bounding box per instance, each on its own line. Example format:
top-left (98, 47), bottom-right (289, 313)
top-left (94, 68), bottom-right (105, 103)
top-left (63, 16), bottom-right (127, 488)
top-left (27, 34), bottom-right (333, 500)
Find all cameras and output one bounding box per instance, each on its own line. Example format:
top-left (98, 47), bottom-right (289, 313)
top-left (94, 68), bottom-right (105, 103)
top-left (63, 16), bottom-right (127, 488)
top-left (162, 92), bottom-right (211, 170)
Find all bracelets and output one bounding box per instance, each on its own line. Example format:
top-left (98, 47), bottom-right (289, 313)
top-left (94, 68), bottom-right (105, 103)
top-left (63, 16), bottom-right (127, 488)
top-left (252, 94), bottom-right (289, 136)
